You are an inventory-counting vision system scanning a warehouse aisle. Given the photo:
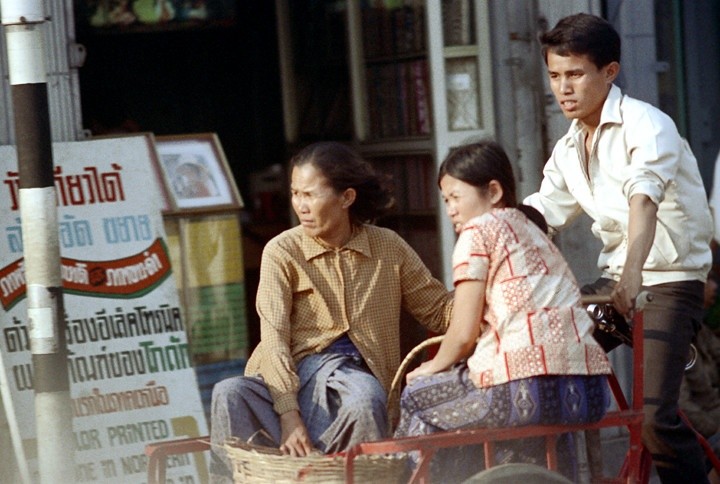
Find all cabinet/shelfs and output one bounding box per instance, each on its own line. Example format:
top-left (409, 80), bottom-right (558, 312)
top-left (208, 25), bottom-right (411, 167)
top-left (277, 0), bottom-right (495, 294)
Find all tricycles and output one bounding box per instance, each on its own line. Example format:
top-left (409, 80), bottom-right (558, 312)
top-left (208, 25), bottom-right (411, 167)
top-left (141, 290), bottom-right (720, 484)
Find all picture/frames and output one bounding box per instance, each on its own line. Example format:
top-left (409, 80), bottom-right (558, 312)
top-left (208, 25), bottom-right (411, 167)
top-left (84, 131), bottom-right (243, 217)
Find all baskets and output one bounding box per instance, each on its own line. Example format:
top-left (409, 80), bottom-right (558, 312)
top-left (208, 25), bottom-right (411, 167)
top-left (221, 444), bottom-right (410, 484)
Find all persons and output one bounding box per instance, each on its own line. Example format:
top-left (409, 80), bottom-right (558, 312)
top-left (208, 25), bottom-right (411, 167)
top-left (173, 153), bottom-right (217, 199)
top-left (392, 139), bottom-right (611, 484)
top-left (210, 143), bottom-right (484, 484)
top-left (523, 13), bottom-right (714, 484)
top-left (702, 153), bottom-right (720, 323)
